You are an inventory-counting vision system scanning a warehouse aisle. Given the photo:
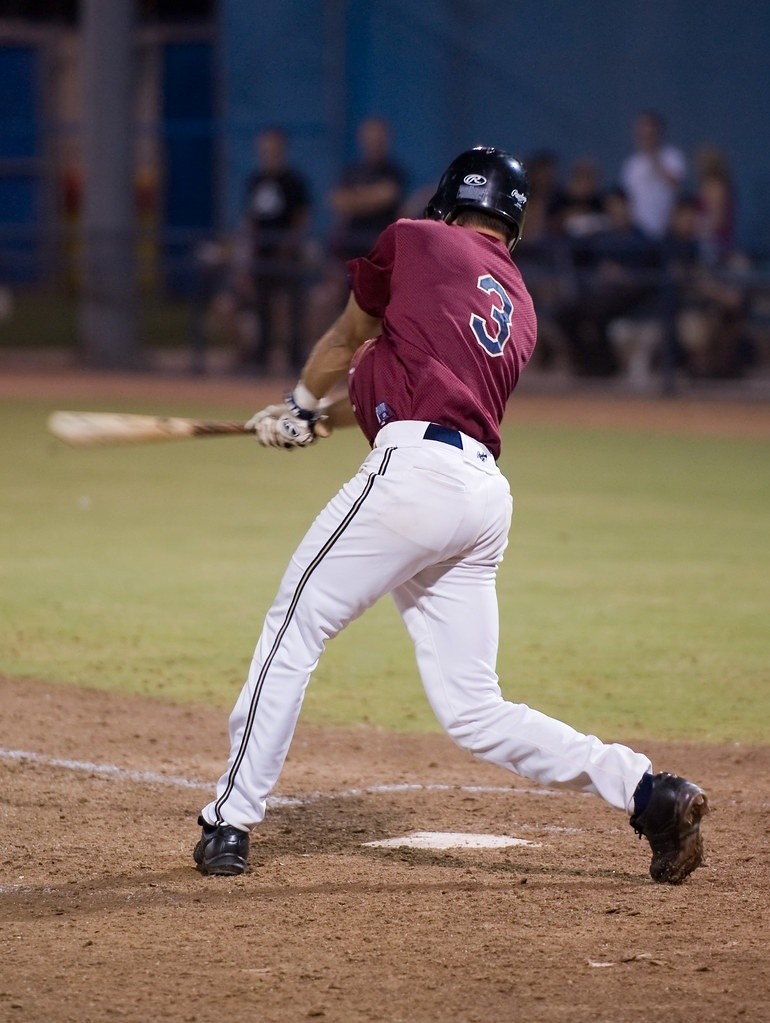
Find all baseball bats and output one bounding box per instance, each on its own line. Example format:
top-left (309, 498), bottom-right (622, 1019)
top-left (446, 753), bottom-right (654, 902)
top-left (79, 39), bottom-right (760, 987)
top-left (47, 410), bottom-right (332, 446)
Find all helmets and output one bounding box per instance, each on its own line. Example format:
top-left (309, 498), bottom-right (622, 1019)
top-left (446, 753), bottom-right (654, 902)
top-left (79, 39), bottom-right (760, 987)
top-left (429, 146), bottom-right (531, 252)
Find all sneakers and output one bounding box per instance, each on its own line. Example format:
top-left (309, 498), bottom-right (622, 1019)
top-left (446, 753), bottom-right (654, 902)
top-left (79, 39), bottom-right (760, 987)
top-left (629, 771), bottom-right (707, 884)
top-left (194, 815), bottom-right (251, 876)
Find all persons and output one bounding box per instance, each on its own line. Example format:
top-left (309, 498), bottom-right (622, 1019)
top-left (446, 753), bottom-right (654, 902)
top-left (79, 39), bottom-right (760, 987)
top-left (192, 144), bottom-right (707, 885)
top-left (232, 105), bottom-right (757, 387)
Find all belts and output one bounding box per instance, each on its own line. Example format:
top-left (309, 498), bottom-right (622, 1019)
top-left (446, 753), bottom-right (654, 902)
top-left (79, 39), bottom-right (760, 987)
top-left (424, 423), bottom-right (463, 450)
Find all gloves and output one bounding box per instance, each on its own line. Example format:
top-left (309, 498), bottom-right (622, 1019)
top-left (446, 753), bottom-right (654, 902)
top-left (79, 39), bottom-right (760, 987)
top-left (243, 381), bottom-right (330, 449)
